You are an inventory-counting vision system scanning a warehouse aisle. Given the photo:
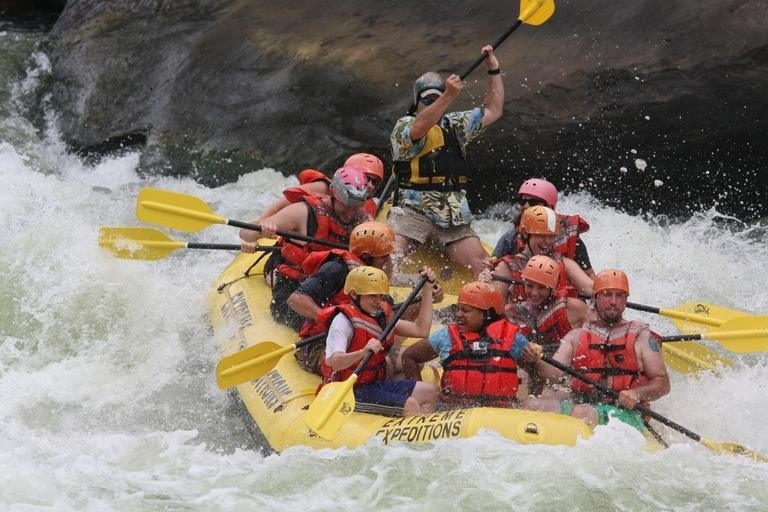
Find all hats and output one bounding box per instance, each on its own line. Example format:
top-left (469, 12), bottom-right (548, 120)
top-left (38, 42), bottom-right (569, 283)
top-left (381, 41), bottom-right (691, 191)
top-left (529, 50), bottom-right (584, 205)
top-left (419, 88), bottom-right (442, 98)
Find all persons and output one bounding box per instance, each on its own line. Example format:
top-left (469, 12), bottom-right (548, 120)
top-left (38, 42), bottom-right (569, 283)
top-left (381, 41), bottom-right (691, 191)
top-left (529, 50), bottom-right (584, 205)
top-left (321, 267), bottom-right (437, 418)
top-left (506, 255), bottom-right (597, 352)
top-left (481, 178), bottom-right (595, 281)
top-left (478, 205), bottom-right (594, 299)
top-left (242, 152), bottom-right (384, 254)
top-left (286, 223), bottom-right (444, 374)
top-left (390, 45), bottom-right (505, 280)
top-left (239, 168), bottom-right (373, 242)
top-left (402, 282), bottom-right (530, 408)
top-left (526, 269), bottom-right (670, 430)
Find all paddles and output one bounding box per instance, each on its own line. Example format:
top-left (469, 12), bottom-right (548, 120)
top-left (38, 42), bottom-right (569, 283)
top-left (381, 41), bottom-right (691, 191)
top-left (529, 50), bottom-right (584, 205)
top-left (216, 331), bottom-right (326, 406)
top-left (542, 352), bottom-right (767, 468)
top-left (303, 273), bottom-right (427, 443)
top-left (136, 187), bottom-right (349, 251)
top-left (491, 272), bottom-right (764, 378)
top-left (459, 0), bottom-right (555, 81)
top-left (99, 226), bottom-right (278, 260)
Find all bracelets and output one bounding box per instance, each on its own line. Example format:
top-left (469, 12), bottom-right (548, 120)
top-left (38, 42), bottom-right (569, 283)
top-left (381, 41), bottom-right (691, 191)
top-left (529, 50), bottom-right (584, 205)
top-left (488, 67), bottom-right (500, 75)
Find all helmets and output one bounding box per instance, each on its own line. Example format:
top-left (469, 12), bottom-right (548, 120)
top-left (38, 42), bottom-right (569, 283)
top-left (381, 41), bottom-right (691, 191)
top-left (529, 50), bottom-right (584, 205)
top-left (343, 266), bottom-right (389, 295)
top-left (517, 178), bottom-right (557, 210)
top-left (592, 269), bottom-right (628, 295)
top-left (520, 255), bottom-right (559, 288)
top-left (413, 71), bottom-right (445, 106)
top-left (456, 281), bottom-right (504, 315)
top-left (329, 166), bottom-right (368, 207)
top-left (518, 205), bottom-right (559, 234)
top-left (348, 221), bottom-right (393, 258)
top-left (343, 152), bottom-right (383, 182)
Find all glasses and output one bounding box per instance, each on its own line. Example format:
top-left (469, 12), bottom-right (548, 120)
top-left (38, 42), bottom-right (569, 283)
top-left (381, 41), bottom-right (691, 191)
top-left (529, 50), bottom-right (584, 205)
top-left (366, 175), bottom-right (379, 186)
top-left (419, 96), bottom-right (431, 106)
top-left (517, 198), bottom-right (543, 206)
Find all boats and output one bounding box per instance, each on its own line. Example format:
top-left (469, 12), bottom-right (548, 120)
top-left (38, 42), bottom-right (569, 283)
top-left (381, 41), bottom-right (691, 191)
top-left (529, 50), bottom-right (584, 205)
top-left (209, 201), bottom-right (667, 456)
